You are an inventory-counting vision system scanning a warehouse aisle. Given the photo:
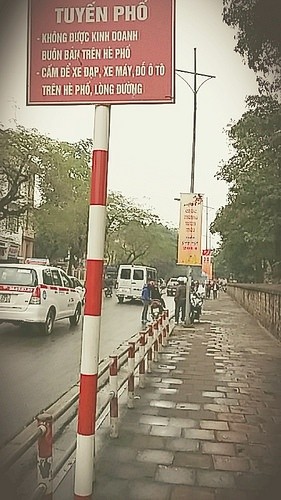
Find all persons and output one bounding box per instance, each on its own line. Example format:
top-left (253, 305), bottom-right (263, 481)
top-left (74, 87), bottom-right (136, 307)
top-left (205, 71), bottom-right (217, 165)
top-left (101, 273), bottom-right (228, 326)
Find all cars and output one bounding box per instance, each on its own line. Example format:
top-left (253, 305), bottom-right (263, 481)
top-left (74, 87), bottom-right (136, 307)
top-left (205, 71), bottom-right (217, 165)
top-left (68, 276), bottom-right (86, 304)
top-left (167, 277), bottom-right (180, 295)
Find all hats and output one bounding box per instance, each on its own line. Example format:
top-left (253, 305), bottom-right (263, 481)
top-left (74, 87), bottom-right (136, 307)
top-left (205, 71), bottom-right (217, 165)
top-left (150, 280), bottom-right (155, 285)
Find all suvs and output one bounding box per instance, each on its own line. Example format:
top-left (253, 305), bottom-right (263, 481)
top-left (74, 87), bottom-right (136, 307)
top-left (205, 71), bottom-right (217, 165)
top-left (1, 264), bottom-right (82, 336)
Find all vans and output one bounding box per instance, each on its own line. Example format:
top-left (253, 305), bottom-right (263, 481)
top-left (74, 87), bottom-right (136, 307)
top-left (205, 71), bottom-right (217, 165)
top-left (116, 265), bottom-right (158, 303)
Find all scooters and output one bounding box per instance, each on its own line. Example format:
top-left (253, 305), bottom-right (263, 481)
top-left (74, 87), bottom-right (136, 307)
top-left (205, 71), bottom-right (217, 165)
top-left (150, 292), bottom-right (166, 321)
top-left (190, 291), bottom-right (204, 323)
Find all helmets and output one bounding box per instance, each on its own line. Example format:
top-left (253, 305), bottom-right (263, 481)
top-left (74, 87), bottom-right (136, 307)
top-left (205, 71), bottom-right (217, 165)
top-left (177, 276), bottom-right (184, 282)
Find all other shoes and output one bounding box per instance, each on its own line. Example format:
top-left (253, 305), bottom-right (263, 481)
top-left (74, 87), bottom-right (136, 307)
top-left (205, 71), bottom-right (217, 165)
top-left (142, 320), bottom-right (150, 323)
top-left (180, 320), bottom-right (184, 324)
top-left (174, 321), bottom-right (178, 325)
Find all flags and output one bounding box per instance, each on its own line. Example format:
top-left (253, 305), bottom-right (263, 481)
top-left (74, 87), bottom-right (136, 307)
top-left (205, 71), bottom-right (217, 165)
top-left (201, 249), bottom-right (213, 281)
top-left (175, 192), bottom-right (205, 268)
